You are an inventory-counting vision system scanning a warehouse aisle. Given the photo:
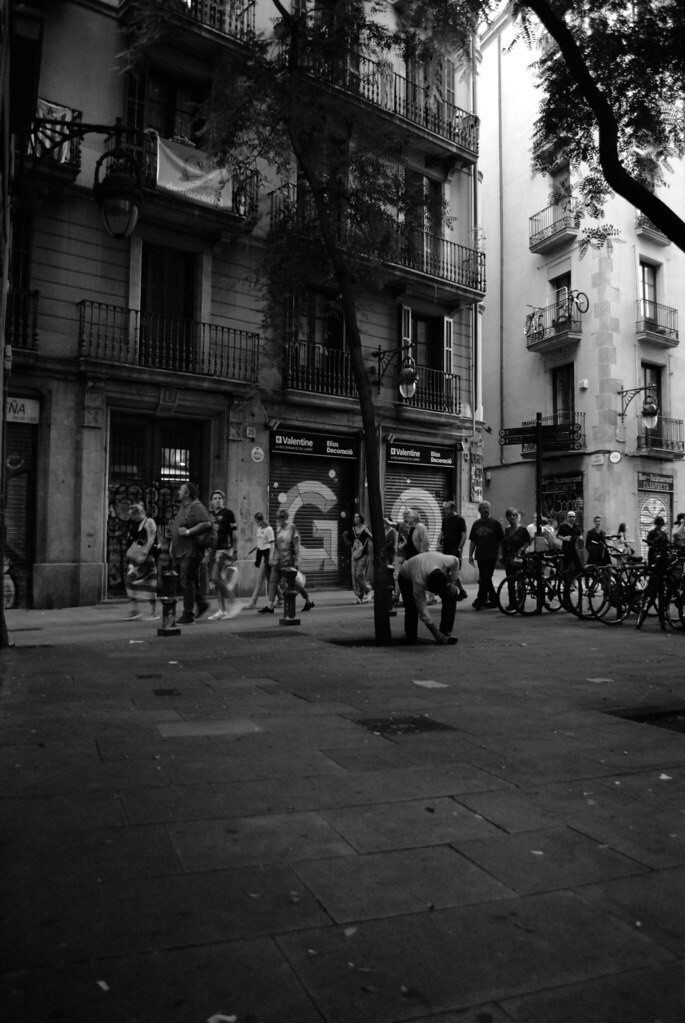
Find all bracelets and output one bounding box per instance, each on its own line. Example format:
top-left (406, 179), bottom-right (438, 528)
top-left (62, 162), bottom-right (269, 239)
top-left (141, 551), bottom-right (148, 556)
top-left (458, 546), bottom-right (462, 553)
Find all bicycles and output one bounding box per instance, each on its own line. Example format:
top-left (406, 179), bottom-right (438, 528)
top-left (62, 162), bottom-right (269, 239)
top-left (494, 536), bottom-right (684, 633)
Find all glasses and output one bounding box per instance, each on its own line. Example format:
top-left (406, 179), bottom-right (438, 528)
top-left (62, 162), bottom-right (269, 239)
top-left (569, 516), bottom-right (575, 518)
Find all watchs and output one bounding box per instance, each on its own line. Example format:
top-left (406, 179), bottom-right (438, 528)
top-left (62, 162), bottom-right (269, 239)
top-left (185, 529), bottom-right (190, 537)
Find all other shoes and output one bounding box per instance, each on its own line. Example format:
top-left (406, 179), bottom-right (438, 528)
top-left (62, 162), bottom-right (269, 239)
top-left (362, 590), bottom-right (374, 603)
top-left (195, 602), bottom-right (209, 618)
top-left (393, 601), bottom-right (404, 607)
top-left (352, 598), bottom-right (362, 604)
top-left (427, 599), bottom-right (437, 605)
top-left (506, 604), bottom-right (516, 611)
top-left (143, 615), bottom-right (160, 620)
top-left (439, 630), bottom-right (458, 643)
top-left (258, 606), bottom-right (275, 615)
top-left (242, 600), bottom-right (256, 609)
top-left (208, 609), bottom-right (228, 620)
top-left (175, 616), bottom-right (195, 626)
top-left (274, 600), bottom-right (285, 608)
top-left (120, 611), bottom-right (143, 621)
top-left (472, 602), bottom-right (481, 610)
top-left (457, 590), bottom-right (467, 601)
top-left (301, 600), bottom-right (315, 612)
top-left (484, 601), bottom-right (497, 608)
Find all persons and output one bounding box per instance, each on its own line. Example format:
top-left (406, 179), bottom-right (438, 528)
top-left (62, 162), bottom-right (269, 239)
top-left (257, 509), bottom-right (315, 613)
top-left (397, 550), bottom-right (460, 645)
top-left (169, 482), bottom-right (213, 626)
top-left (383, 509), bottom-right (437, 605)
top-left (527, 510), bottom-right (632, 594)
top-left (209, 492), bottom-right (243, 620)
top-left (241, 513), bottom-right (285, 609)
top-left (120, 501), bottom-right (161, 621)
top-left (647, 513), bottom-right (685, 575)
top-left (468, 502), bottom-right (505, 609)
top-left (342, 512), bottom-right (374, 605)
top-left (499, 507), bottom-right (531, 612)
top-left (434, 501), bottom-right (466, 601)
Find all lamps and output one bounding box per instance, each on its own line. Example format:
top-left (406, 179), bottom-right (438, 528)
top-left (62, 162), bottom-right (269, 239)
top-left (375, 340), bottom-right (420, 398)
top-left (92, 116), bottom-right (140, 240)
top-left (615, 381), bottom-right (659, 429)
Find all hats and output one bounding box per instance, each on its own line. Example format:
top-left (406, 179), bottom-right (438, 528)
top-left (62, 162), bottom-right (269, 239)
top-left (673, 513), bottom-right (685, 524)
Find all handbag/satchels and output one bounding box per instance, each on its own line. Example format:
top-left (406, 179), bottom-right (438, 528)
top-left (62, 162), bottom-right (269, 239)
top-left (126, 539), bottom-right (145, 561)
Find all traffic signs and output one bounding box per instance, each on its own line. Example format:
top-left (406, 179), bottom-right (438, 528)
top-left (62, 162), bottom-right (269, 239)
top-left (498, 422), bottom-right (582, 451)
